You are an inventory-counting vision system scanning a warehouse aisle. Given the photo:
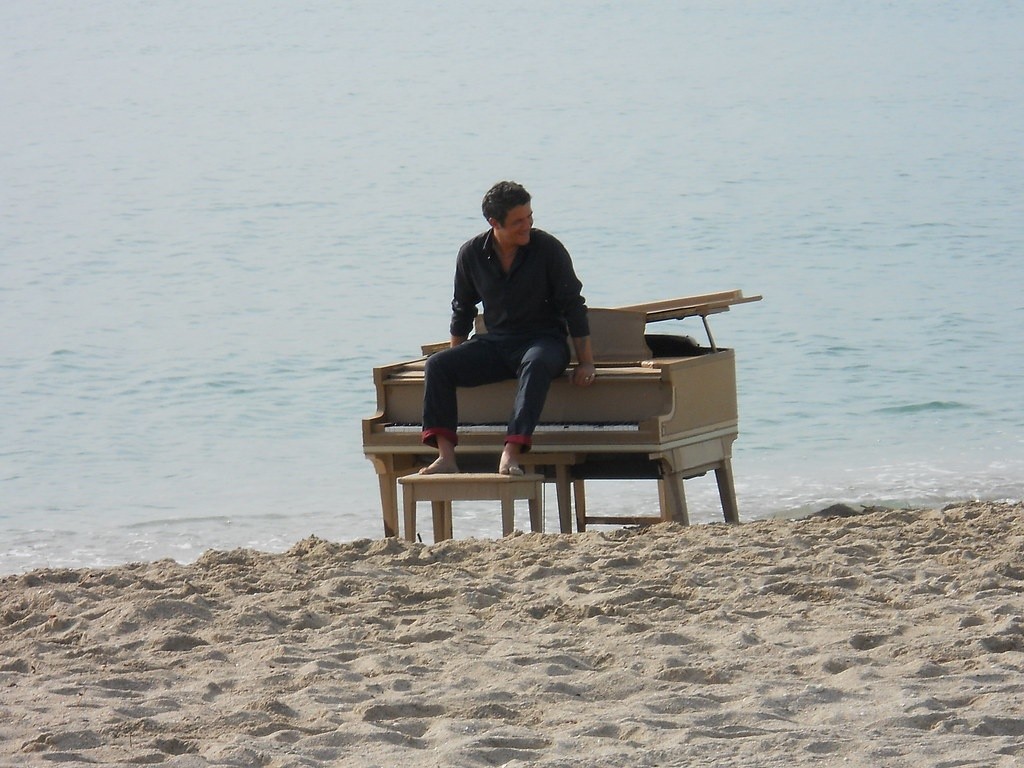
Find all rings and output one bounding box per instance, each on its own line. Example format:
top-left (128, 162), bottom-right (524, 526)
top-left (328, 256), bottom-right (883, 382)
top-left (585, 377), bottom-right (590, 380)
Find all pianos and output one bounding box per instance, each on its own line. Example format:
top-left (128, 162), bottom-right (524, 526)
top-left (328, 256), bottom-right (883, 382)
top-left (359, 288), bottom-right (765, 541)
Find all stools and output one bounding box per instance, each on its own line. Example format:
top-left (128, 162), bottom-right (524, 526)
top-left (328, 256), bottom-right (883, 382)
top-left (400, 468), bottom-right (547, 543)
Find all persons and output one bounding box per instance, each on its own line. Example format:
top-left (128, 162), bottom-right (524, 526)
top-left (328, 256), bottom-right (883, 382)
top-left (418, 181), bottom-right (595, 477)
